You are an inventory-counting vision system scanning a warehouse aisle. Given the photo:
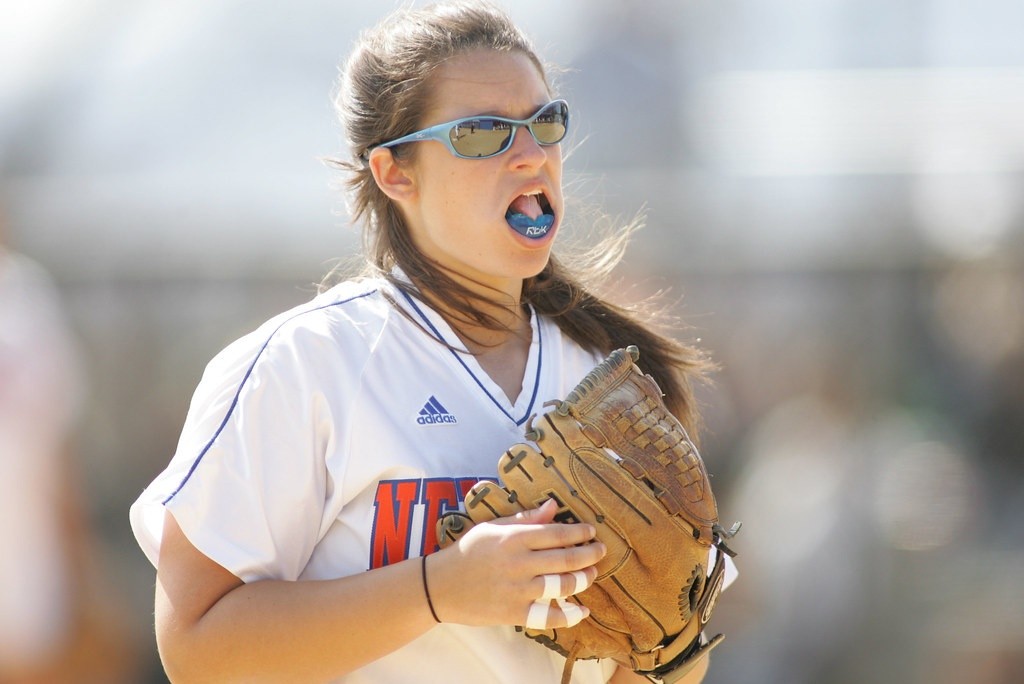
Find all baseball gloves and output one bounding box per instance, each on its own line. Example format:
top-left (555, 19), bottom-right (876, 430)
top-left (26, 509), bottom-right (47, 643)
top-left (433, 343), bottom-right (744, 683)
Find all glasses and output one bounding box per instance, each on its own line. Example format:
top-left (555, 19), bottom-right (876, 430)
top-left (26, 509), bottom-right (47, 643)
top-left (359, 99), bottom-right (569, 162)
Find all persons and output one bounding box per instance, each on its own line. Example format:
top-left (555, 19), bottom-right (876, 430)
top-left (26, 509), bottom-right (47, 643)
top-left (127, 3), bottom-right (740, 684)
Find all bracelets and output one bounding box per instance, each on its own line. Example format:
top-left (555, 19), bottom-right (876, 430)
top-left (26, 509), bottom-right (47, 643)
top-left (422, 555), bottom-right (442, 623)
